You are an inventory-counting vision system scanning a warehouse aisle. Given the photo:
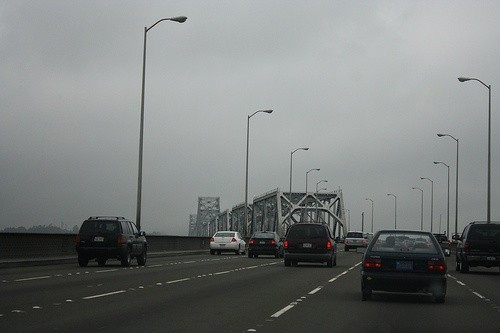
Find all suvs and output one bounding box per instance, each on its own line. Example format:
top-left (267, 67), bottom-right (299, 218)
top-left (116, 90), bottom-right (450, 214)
top-left (451, 220), bottom-right (500, 273)
top-left (78, 216), bottom-right (147, 266)
top-left (282, 220), bottom-right (340, 268)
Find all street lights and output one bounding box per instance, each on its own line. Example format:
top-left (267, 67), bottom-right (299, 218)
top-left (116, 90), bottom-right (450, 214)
top-left (136, 15), bottom-right (188, 229)
top-left (457, 77), bottom-right (491, 222)
top-left (421, 178), bottom-right (434, 234)
top-left (243, 109), bottom-right (273, 234)
top-left (387, 193), bottom-right (397, 229)
top-left (437, 133), bottom-right (458, 235)
top-left (289, 147), bottom-right (309, 227)
top-left (366, 197), bottom-right (373, 232)
top-left (412, 186), bottom-right (423, 230)
top-left (433, 161), bottom-right (449, 239)
top-left (305, 168), bottom-right (328, 223)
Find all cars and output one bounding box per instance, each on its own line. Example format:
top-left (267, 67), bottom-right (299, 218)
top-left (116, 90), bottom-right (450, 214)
top-left (209, 230), bottom-right (246, 255)
top-left (248, 231), bottom-right (284, 259)
top-left (344, 231), bottom-right (462, 255)
top-left (360, 230), bottom-right (447, 303)
